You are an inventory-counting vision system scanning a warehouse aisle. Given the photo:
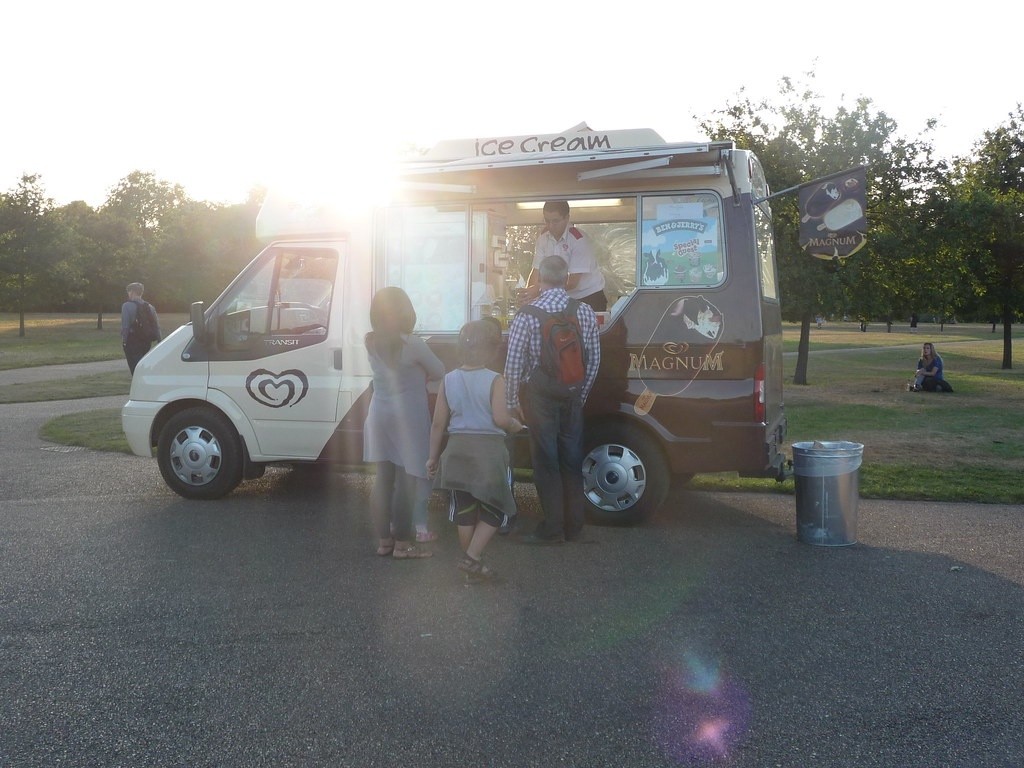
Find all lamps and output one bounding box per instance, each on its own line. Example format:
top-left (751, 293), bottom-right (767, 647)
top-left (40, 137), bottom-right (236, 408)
top-left (516, 199), bottom-right (624, 209)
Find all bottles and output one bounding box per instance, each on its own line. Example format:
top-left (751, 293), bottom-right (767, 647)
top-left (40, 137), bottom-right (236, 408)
top-left (491, 303), bottom-right (501, 322)
top-left (507, 303), bottom-right (517, 328)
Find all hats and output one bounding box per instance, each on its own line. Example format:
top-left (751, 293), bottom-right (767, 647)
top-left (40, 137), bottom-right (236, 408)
top-left (454, 316), bottom-right (502, 367)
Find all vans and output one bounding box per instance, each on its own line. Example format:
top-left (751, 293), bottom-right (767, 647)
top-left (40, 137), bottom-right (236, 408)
top-left (118, 124), bottom-right (792, 525)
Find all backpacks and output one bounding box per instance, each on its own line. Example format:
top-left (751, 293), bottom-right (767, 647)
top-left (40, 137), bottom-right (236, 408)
top-left (127, 300), bottom-right (159, 341)
top-left (517, 298), bottom-right (589, 400)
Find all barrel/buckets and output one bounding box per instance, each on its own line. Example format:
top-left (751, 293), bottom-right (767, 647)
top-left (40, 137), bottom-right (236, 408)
top-left (790, 439), bottom-right (865, 546)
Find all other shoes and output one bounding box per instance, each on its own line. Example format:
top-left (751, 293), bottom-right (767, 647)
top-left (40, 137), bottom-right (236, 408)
top-left (415, 531), bottom-right (438, 543)
top-left (564, 531), bottom-right (583, 543)
top-left (516, 530), bottom-right (566, 546)
top-left (392, 544), bottom-right (433, 560)
top-left (377, 546), bottom-right (393, 556)
top-left (915, 383), bottom-right (922, 392)
top-left (906, 385), bottom-right (917, 392)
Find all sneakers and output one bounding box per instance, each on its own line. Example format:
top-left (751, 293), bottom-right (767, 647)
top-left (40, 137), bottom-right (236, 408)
top-left (457, 553), bottom-right (501, 582)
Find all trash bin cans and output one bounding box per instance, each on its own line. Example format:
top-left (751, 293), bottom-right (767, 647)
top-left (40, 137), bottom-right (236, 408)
top-left (791, 441), bottom-right (864, 547)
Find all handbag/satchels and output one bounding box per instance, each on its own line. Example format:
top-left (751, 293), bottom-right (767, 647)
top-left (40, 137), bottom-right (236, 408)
top-left (526, 363), bottom-right (572, 413)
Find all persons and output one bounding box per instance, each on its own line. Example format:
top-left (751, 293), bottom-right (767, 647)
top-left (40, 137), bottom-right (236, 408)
top-left (859, 315), bottom-right (865, 321)
top-left (815, 314), bottom-right (822, 328)
top-left (120, 281), bottom-right (163, 372)
top-left (514, 200), bottom-right (608, 311)
top-left (502, 256), bottom-right (601, 543)
top-left (426, 317), bottom-right (522, 576)
top-left (449, 315), bottom-right (515, 536)
top-left (907, 342), bottom-right (955, 392)
top-left (363, 287), bottom-right (445, 558)
top-left (390, 472), bottom-right (438, 542)
